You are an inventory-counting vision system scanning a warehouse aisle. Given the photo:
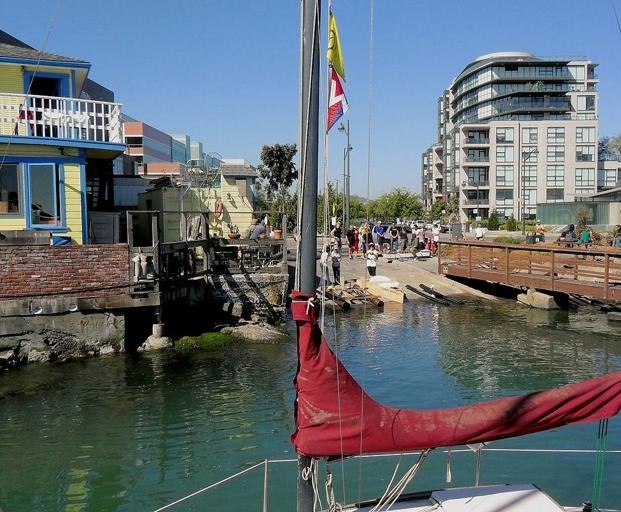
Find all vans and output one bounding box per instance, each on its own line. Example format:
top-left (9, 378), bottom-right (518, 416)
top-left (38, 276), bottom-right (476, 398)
top-left (371, 225), bottom-right (433, 250)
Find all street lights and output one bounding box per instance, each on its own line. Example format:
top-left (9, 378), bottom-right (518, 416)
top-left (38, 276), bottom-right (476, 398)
top-left (522, 148), bottom-right (539, 234)
top-left (337, 120), bottom-right (353, 239)
top-left (462, 180), bottom-right (480, 216)
top-left (441, 208), bottom-right (445, 225)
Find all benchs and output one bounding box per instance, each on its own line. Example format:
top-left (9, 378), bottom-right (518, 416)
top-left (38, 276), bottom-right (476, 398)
top-left (556, 239), bottom-right (594, 250)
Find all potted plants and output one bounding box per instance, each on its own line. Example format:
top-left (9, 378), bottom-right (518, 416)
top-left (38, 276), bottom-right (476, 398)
top-left (267, 200), bottom-right (283, 240)
top-left (227, 222), bottom-right (241, 239)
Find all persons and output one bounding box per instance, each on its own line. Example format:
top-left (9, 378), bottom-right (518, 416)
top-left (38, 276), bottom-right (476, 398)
top-left (475, 223), bottom-right (484, 241)
top-left (560, 224), bottom-right (621, 247)
top-left (246, 210), bottom-right (458, 286)
top-left (534, 219), bottom-right (544, 235)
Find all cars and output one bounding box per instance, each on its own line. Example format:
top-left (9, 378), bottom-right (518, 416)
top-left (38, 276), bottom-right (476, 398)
top-left (407, 220), bottom-right (433, 231)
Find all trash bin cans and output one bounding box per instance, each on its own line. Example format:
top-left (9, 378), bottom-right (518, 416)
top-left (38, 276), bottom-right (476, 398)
top-left (452, 223), bottom-right (463, 240)
top-left (526, 231), bottom-right (545, 244)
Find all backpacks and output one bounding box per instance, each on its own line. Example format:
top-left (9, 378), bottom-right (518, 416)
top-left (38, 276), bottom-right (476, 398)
top-left (331, 256), bottom-right (339, 268)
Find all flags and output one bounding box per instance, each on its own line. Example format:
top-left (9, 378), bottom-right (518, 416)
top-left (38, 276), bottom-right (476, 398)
top-left (327, 4), bottom-right (348, 83)
top-left (326, 65), bottom-right (351, 134)
